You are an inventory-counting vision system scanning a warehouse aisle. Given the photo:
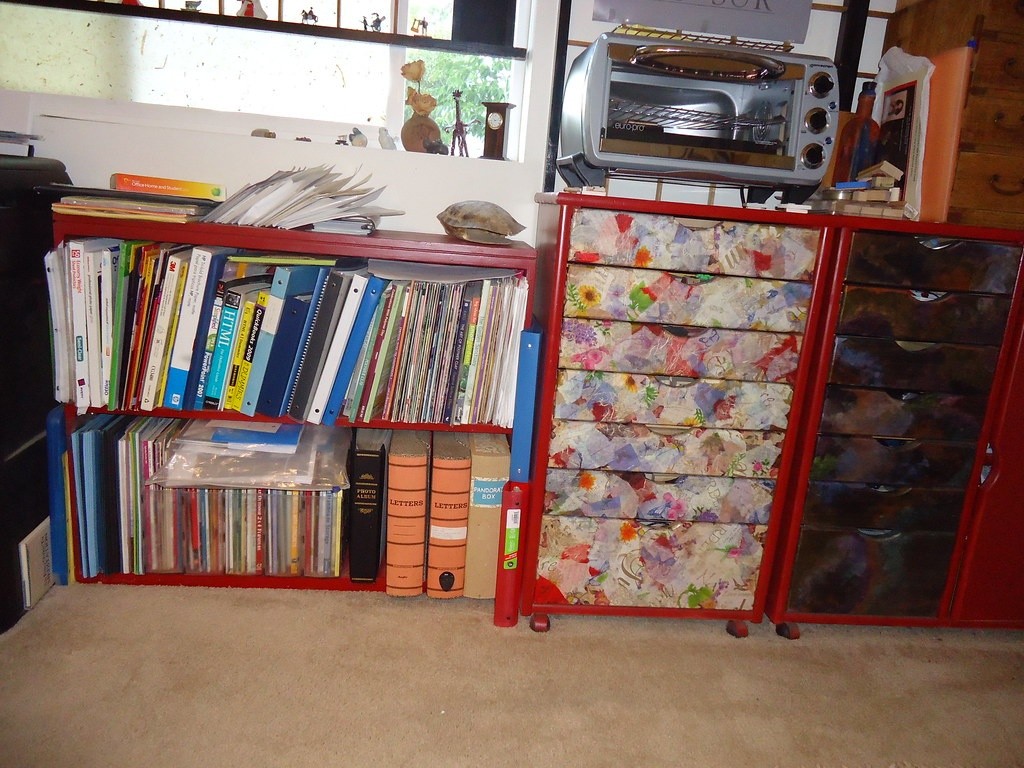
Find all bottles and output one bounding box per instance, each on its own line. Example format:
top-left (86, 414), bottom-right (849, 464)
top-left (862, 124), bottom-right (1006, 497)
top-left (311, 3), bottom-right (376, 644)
top-left (831, 81), bottom-right (879, 185)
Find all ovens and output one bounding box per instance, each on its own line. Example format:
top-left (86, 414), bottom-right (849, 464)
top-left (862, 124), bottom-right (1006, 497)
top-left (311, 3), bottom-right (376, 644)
top-left (553, 22), bottom-right (841, 215)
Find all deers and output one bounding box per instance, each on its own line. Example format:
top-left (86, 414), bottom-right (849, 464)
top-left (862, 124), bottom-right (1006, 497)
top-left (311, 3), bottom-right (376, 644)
top-left (449, 90), bottom-right (469, 157)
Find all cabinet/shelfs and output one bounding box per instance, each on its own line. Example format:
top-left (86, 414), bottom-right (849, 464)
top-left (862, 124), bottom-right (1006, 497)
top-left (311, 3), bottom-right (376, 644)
top-left (880, 1), bottom-right (1024, 223)
top-left (521, 191), bottom-right (833, 639)
top-left (49, 203), bottom-right (538, 591)
top-left (764, 214), bottom-right (1023, 639)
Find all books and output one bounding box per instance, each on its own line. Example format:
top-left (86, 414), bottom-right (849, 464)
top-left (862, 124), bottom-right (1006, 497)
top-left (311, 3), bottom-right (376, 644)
top-left (462, 431), bottom-right (513, 600)
top-left (350, 428), bottom-right (392, 580)
top-left (42, 238), bottom-right (530, 431)
top-left (921, 45), bottom-right (970, 225)
top-left (387, 431), bottom-right (432, 597)
top-left (32, 161), bottom-right (404, 235)
top-left (425, 432), bottom-right (472, 598)
top-left (18, 513), bottom-right (55, 611)
top-left (71, 416), bottom-right (351, 578)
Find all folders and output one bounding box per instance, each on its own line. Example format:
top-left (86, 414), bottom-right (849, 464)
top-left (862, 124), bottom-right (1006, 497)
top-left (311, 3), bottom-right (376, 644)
top-left (18, 160), bottom-right (539, 610)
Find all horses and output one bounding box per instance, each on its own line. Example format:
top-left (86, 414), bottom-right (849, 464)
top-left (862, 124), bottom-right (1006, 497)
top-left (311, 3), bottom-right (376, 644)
top-left (301, 10), bottom-right (318, 26)
